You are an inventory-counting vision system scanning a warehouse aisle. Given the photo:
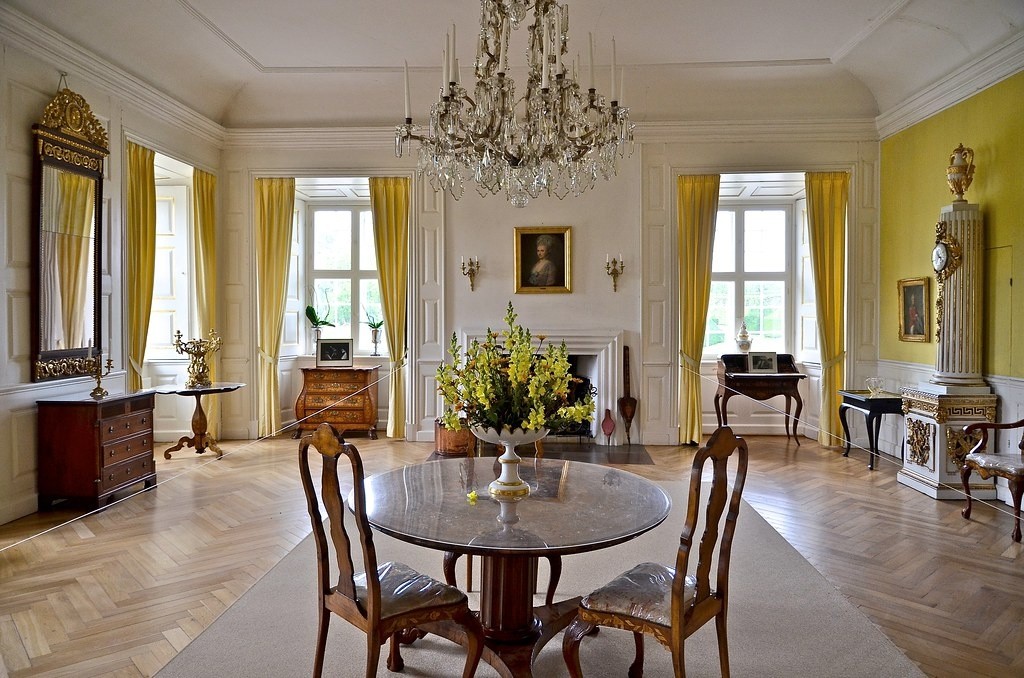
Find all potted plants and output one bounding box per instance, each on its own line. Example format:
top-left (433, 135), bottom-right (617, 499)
top-left (303, 282), bottom-right (334, 354)
top-left (359, 305), bottom-right (384, 356)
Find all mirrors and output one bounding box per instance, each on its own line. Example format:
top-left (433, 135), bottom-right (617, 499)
top-left (32, 86), bottom-right (112, 385)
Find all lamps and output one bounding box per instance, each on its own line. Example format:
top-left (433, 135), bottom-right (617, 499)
top-left (395, 0), bottom-right (636, 208)
top-left (462, 255), bottom-right (480, 291)
top-left (606, 253), bottom-right (625, 291)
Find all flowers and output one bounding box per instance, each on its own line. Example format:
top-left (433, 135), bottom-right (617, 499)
top-left (433, 301), bottom-right (596, 435)
top-left (466, 491), bottom-right (478, 503)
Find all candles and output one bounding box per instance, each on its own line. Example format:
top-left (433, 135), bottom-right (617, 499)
top-left (108, 337), bottom-right (112, 360)
top-left (88, 338), bottom-right (92, 359)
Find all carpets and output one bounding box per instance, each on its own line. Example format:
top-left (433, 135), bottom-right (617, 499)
top-left (152, 483), bottom-right (927, 678)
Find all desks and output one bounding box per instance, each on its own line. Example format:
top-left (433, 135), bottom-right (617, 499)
top-left (837, 389), bottom-right (905, 470)
top-left (152, 382), bottom-right (248, 461)
top-left (350, 457), bottom-right (671, 678)
top-left (714, 354), bottom-right (808, 446)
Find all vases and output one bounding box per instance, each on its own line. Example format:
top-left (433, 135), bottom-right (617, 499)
top-left (468, 425), bottom-right (550, 499)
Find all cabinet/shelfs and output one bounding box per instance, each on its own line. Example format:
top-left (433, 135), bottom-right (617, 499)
top-left (290, 367), bottom-right (380, 440)
top-left (34, 390), bottom-right (158, 511)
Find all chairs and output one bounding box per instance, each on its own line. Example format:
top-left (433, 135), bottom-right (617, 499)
top-left (299, 422), bottom-right (484, 678)
top-left (960, 418), bottom-right (1024, 542)
top-left (562, 426), bottom-right (750, 678)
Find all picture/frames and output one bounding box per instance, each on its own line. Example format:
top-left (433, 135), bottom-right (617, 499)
top-left (316, 339), bottom-right (353, 368)
top-left (472, 460), bottom-right (571, 503)
top-left (897, 276), bottom-right (930, 342)
top-left (748, 352), bottom-right (778, 374)
top-left (513, 227), bottom-right (572, 294)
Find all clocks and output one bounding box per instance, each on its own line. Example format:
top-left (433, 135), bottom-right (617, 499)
top-left (932, 222), bottom-right (962, 282)
